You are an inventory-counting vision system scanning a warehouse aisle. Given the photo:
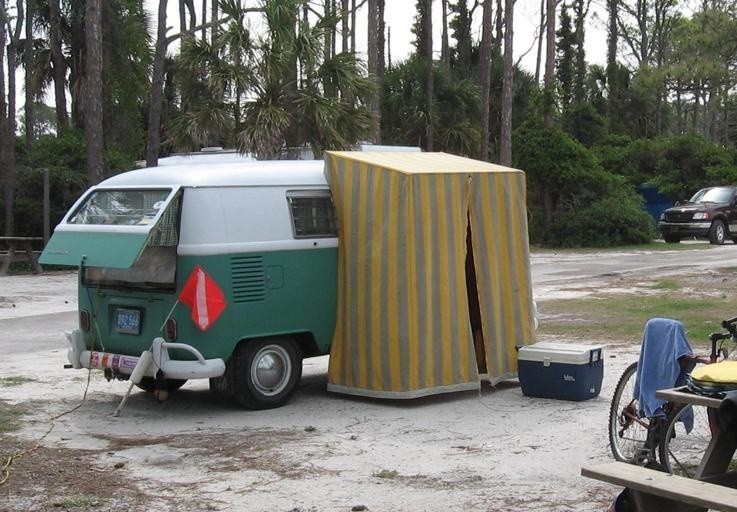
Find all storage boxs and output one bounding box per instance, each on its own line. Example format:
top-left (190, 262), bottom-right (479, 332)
top-left (514, 340), bottom-right (603, 402)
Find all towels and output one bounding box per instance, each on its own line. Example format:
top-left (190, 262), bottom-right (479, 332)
top-left (632, 318), bottom-right (694, 435)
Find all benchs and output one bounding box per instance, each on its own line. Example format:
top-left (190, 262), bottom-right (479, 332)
top-left (580, 460), bottom-right (736, 511)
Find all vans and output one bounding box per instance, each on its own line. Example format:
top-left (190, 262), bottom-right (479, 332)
top-left (36, 159), bottom-right (339, 409)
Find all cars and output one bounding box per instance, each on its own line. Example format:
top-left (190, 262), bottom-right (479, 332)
top-left (658, 186), bottom-right (737, 245)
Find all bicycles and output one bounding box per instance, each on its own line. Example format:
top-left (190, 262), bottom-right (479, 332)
top-left (608, 317), bottom-right (737, 476)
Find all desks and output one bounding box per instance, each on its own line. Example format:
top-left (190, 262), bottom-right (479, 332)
top-left (655, 383), bottom-right (736, 481)
